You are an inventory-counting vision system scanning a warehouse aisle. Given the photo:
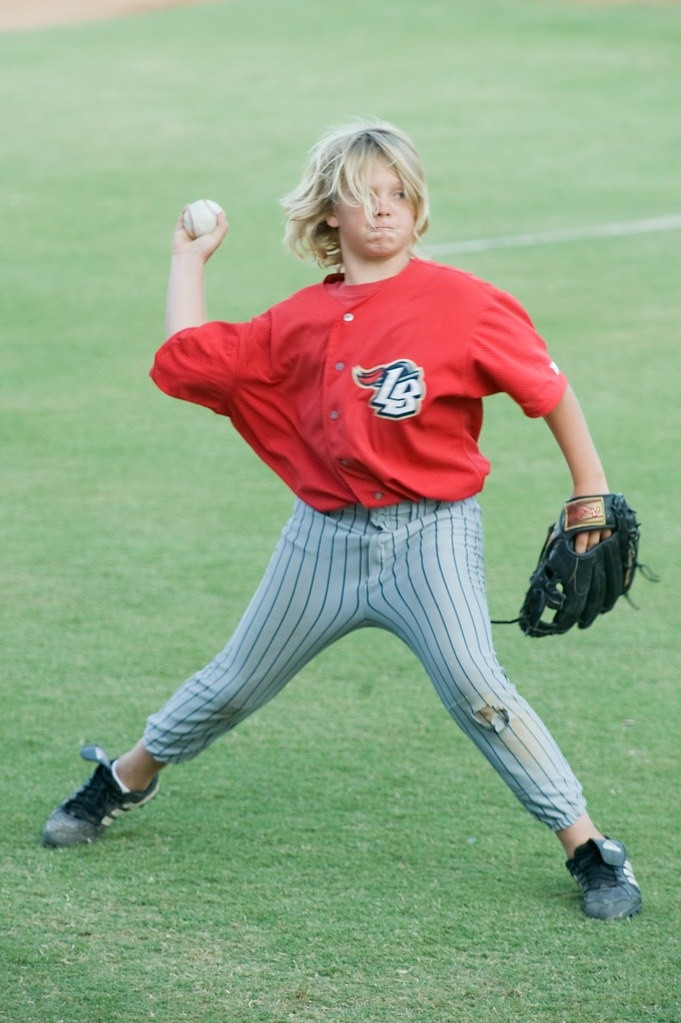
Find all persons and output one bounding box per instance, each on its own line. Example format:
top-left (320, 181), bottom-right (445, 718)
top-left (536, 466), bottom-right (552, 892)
top-left (43, 112), bottom-right (645, 924)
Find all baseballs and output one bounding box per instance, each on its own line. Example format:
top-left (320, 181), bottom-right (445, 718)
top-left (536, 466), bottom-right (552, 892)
top-left (184, 200), bottom-right (224, 239)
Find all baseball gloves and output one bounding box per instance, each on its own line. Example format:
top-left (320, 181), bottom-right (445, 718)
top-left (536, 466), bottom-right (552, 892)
top-left (518, 493), bottom-right (638, 637)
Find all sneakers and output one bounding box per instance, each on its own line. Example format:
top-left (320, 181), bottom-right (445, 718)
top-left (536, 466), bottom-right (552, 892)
top-left (563, 834), bottom-right (644, 922)
top-left (41, 746), bottom-right (160, 849)
